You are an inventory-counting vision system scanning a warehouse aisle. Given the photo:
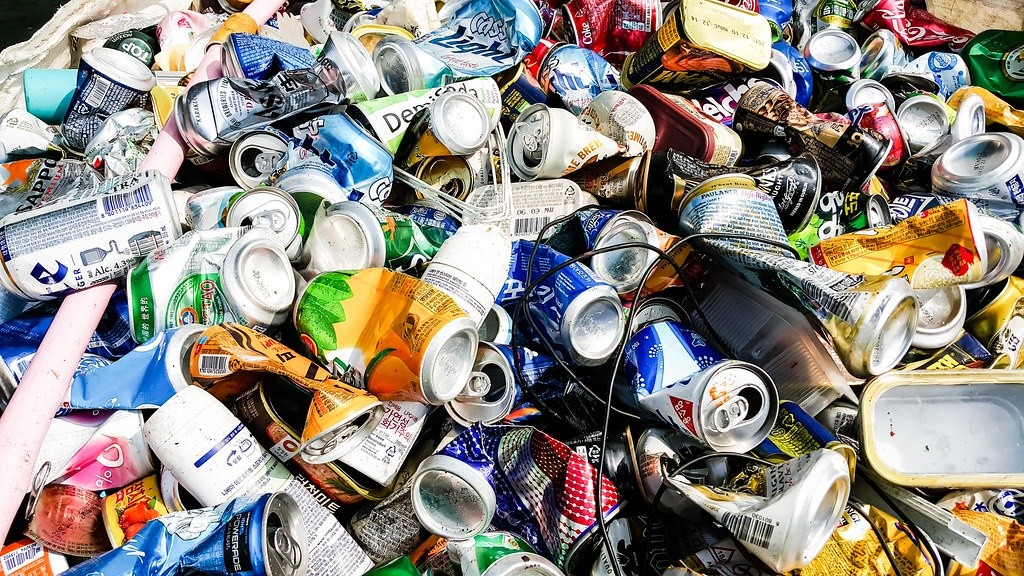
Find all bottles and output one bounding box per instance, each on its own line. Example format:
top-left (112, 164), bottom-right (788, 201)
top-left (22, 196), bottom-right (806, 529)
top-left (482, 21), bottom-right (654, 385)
top-left (141, 384), bottom-right (377, 576)
top-left (338, 222), bottom-right (513, 490)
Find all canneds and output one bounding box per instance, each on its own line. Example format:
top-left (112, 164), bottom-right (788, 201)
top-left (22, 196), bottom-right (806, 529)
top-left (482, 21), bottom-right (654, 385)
top-left (0, 0), bottom-right (1024, 576)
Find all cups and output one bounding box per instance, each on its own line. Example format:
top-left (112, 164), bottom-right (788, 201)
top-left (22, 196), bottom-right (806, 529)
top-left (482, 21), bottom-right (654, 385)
top-left (23, 69), bottom-right (78, 124)
